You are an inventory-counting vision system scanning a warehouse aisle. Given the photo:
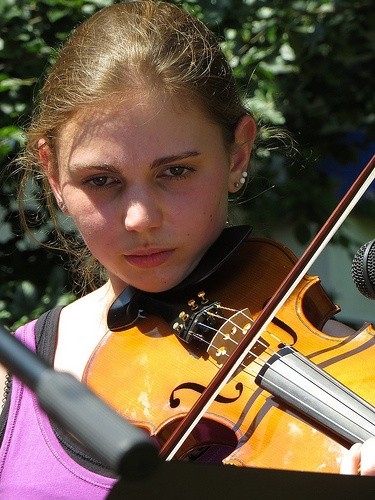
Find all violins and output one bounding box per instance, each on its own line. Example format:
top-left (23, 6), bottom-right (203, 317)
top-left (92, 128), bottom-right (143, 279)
top-left (84, 225), bottom-right (374, 472)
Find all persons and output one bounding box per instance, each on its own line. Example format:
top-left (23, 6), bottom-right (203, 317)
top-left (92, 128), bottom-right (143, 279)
top-left (0, 0), bottom-right (375, 500)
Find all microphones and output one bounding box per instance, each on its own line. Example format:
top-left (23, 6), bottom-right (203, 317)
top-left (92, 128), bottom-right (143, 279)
top-left (0, 325), bottom-right (156, 476)
top-left (350, 241), bottom-right (375, 300)
